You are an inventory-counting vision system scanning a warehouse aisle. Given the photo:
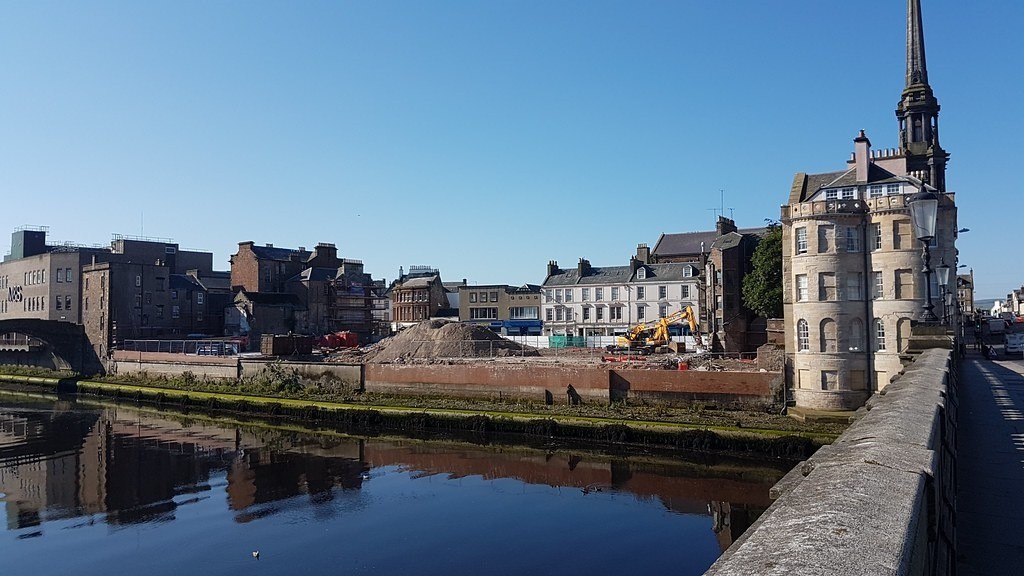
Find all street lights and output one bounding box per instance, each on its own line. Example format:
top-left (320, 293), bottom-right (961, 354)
top-left (944, 289), bottom-right (954, 325)
top-left (933, 255), bottom-right (951, 325)
top-left (904, 180), bottom-right (942, 326)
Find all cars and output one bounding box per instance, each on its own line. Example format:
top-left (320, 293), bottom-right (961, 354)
top-left (196, 342), bottom-right (238, 356)
top-left (1002, 332), bottom-right (1024, 356)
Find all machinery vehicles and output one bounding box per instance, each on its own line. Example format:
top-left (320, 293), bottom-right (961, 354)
top-left (606, 304), bottom-right (707, 357)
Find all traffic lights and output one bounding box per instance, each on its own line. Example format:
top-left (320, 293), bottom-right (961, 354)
top-left (975, 318), bottom-right (982, 333)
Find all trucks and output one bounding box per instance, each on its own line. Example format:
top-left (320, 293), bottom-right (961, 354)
top-left (976, 310), bottom-right (1024, 337)
